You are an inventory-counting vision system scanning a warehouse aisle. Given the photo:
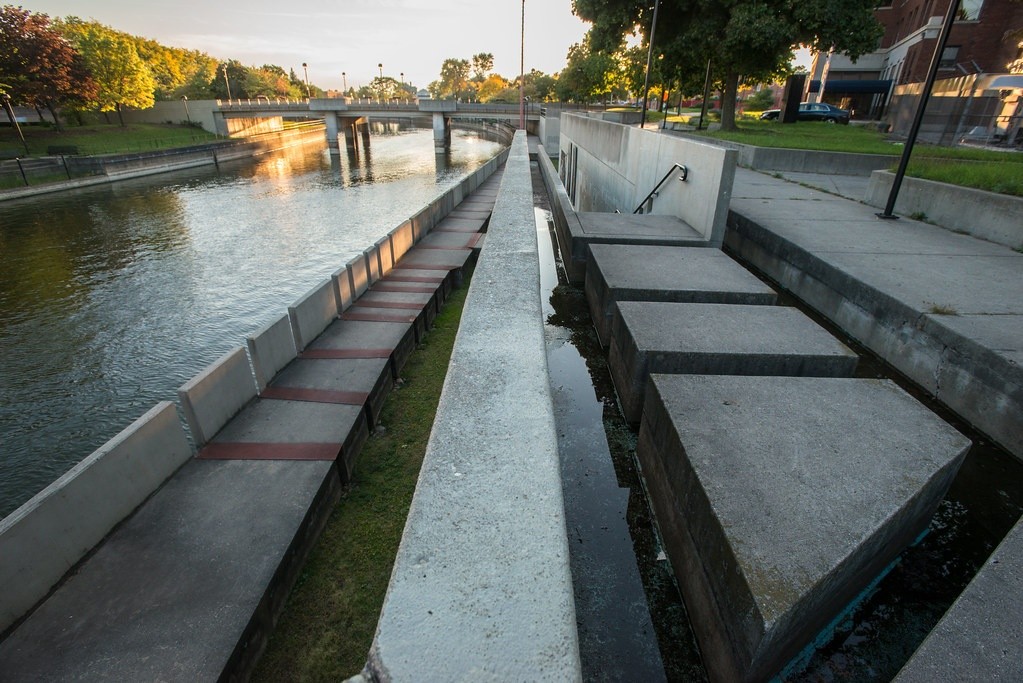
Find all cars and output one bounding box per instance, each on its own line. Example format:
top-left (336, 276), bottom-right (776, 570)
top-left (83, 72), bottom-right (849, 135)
top-left (759, 101), bottom-right (851, 126)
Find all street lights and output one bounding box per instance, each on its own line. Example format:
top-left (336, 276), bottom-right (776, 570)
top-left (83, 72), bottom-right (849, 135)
top-left (378, 63), bottom-right (383, 92)
top-left (342, 72), bottom-right (348, 99)
top-left (180, 94), bottom-right (191, 126)
top-left (303, 62), bottom-right (311, 99)
top-left (400, 73), bottom-right (404, 86)
